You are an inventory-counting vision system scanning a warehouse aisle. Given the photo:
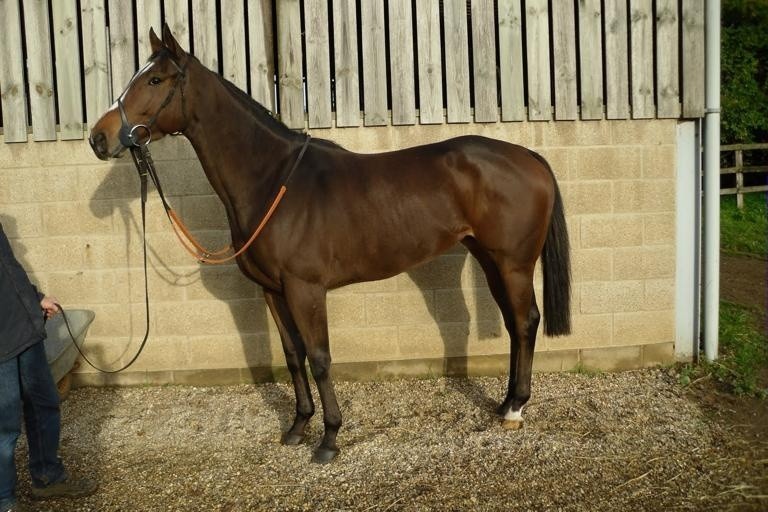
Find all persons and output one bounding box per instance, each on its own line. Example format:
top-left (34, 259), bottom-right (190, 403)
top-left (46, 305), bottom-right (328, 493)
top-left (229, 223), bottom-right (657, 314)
top-left (1, 223), bottom-right (99, 511)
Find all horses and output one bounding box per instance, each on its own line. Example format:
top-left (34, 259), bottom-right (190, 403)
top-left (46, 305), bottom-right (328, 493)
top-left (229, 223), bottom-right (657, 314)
top-left (88, 21), bottom-right (571, 464)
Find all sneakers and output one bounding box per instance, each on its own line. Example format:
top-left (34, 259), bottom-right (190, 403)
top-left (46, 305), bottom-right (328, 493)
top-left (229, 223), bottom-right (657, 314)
top-left (30, 474), bottom-right (99, 500)
